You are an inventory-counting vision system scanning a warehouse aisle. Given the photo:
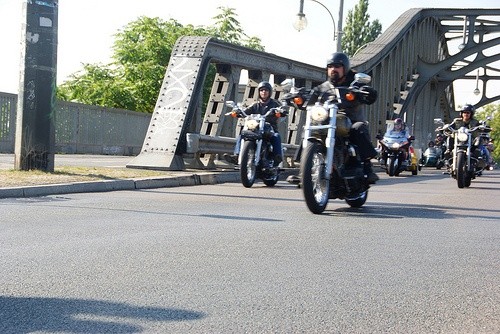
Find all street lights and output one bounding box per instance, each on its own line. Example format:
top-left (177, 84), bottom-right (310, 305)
top-left (293, 0), bottom-right (345, 52)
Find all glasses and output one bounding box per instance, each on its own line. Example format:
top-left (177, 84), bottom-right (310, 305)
top-left (260, 88), bottom-right (269, 91)
top-left (463, 111), bottom-right (470, 114)
top-left (328, 64), bottom-right (340, 68)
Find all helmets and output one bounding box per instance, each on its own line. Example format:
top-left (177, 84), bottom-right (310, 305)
top-left (459, 104), bottom-right (475, 116)
top-left (394, 118), bottom-right (403, 128)
top-left (257, 83), bottom-right (272, 97)
top-left (327, 53), bottom-right (350, 76)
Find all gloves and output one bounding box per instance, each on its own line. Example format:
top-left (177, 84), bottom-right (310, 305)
top-left (484, 126), bottom-right (492, 133)
top-left (359, 87), bottom-right (377, 105)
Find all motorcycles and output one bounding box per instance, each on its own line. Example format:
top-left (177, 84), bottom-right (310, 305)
top-left (377, 118), bottom-right (494, 189)
top-left (280, 71), bottom-right (372, 214)
top-left (224, 95), bottom-right (289, 189)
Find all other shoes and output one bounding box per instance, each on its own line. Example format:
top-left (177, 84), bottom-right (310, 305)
top-left (286, 172), bottom-right (300, 185)
top-left (274, 154), bottom-right (281, 167)
top-left (362, 165), bottom-right (379, 182)
top-left (223, 152), bottom-right (238, 164)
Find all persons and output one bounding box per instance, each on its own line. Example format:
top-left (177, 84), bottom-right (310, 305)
top-left (285, 53), bottom-right (379, 183)
top-left (376, 118), bottom-right (412, 161)
top-left (235, 82), bottom-right (287, 174)
top-left (438, 104), bottom-right (486, 174)
top-left (427, 133), bottom-right (445, 147)
